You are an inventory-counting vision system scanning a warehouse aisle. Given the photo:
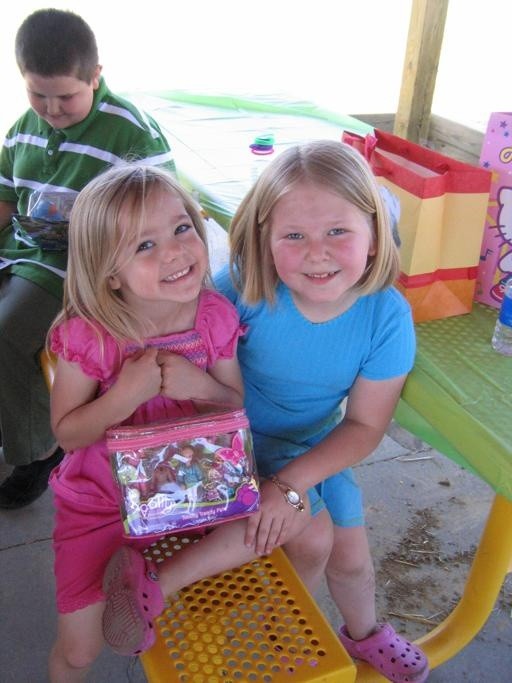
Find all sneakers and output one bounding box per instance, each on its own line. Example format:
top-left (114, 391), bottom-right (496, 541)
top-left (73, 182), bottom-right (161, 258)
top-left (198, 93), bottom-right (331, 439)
top-left (0, 446), bottom-right (65, 509)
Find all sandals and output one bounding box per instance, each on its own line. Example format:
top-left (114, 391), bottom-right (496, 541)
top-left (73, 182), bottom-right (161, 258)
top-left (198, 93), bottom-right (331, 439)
top-left (101, 547), bottom-right (168, 660)
top-left (338, 618), bottom-right (430, 683)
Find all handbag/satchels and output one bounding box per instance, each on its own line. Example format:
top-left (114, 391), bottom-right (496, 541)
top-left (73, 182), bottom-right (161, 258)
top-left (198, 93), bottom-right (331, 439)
top-left (341, 124), bottom-right (493, 323)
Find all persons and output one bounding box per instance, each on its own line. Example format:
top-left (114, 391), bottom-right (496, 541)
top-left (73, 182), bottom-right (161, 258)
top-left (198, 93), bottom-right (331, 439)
top-left (205, 138), bottom-right (430, 683)
top-left (0, 8), bottom-right (178, 511)
top-left (46, 163), bottom-right (310, 683)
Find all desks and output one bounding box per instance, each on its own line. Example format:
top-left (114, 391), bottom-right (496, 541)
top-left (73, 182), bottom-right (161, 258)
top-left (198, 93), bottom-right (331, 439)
top-left (391, 299), bottom-right (512, 503)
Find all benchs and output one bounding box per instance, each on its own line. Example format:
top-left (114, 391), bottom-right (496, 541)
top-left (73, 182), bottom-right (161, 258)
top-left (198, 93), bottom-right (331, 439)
top-left (38, 344), bottom-right (357, 683)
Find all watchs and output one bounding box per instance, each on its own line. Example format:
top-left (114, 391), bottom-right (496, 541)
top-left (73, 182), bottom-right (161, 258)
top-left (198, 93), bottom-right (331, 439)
top-left (270, 476), bottom-right (305, 512)
